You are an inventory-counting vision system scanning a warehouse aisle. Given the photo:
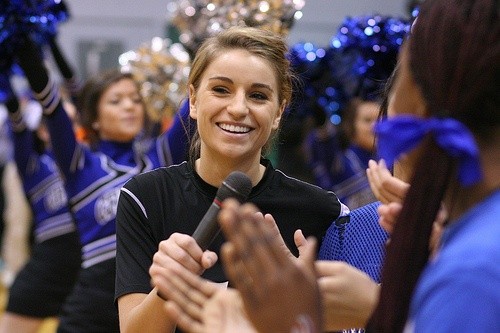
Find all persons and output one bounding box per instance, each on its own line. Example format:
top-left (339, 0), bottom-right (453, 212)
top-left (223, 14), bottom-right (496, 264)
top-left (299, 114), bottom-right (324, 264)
top-left (329, 95), bottom-right (387, 210)
top-left (15, 34), bottom-right (196, 333)
top-left (148, 0), bottom-right (500, 333)
top-left (0, 72), bottom-right (84, 333)
top-left (114, 26), bottom-right (350, 333)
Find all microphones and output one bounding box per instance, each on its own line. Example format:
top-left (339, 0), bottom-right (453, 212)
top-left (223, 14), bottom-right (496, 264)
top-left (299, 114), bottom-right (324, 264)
top-left (156, 172), bottom-right (253, 301)
